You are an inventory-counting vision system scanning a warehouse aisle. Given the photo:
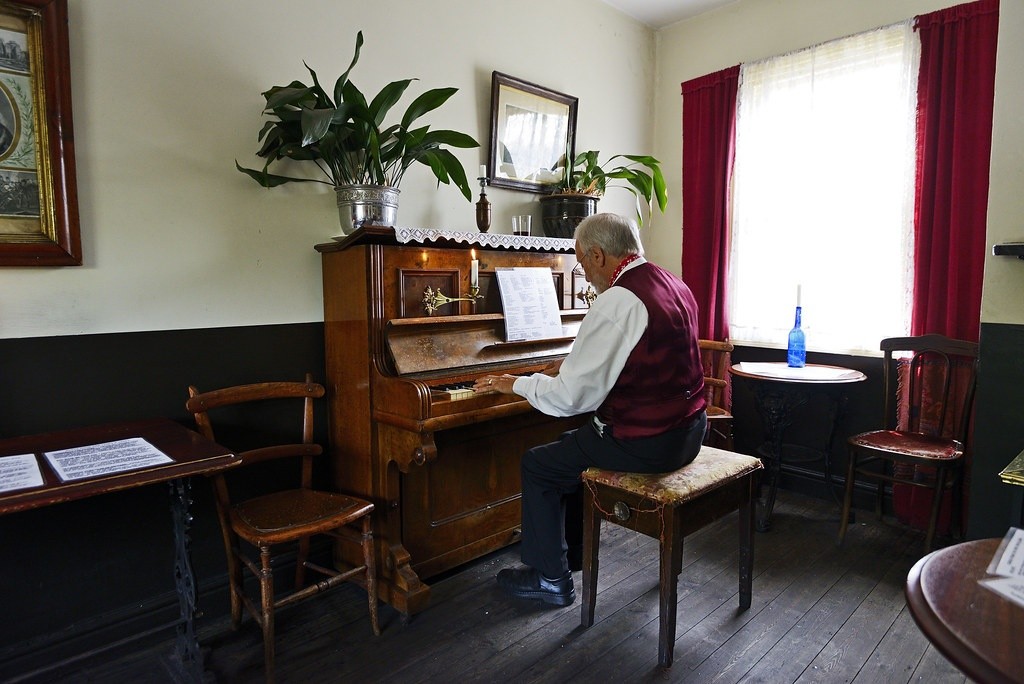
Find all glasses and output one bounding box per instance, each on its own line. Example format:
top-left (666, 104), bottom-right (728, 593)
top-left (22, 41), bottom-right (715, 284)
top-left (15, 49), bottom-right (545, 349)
top-left (570, 248), bottom-right (593, 277)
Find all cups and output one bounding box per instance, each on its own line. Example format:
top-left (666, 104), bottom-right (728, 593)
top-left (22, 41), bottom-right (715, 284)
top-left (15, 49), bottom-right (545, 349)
top-left (511, 214), bottom-right (532, 236)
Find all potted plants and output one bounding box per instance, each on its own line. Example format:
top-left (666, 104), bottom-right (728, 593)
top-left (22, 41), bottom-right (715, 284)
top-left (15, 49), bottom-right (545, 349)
top-left (235, 30), bottom-right (480, 235)
top-left (540, 150), bottom-right (668, 238)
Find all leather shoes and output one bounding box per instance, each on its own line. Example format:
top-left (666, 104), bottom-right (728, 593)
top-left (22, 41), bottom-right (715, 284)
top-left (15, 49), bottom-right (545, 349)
top-left (496, 567), bottom-right (576, 606)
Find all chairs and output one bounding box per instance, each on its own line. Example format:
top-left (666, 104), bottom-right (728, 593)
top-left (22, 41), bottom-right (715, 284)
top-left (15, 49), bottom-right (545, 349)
top-left (187, 373), bottom-right (382, 684)
top-left (699, 340), bottom-right (734, 449)
top-left (838, 335), bottom-right (982, 555)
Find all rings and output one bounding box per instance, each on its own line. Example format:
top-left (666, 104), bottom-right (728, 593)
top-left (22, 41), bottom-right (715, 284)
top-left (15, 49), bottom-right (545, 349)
top-left (489, 378), bottom-right (492, 385)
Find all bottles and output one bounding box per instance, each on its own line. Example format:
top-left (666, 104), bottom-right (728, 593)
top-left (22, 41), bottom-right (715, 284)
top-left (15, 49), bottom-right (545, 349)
top-left (787, 306), bottom-right (806, 368)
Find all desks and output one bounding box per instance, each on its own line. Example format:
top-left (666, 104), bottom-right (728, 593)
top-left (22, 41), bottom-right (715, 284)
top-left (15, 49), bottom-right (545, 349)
top-left (728, 362), bottom-right (868, 534)
top-left (0, 419), bottom-right (241, 684)
top-left (904, 539), bottom-right (1024, 684)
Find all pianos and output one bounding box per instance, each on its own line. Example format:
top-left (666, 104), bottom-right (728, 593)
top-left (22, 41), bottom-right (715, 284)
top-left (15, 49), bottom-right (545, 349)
top-left (314, 226), bottom-right (600, 628)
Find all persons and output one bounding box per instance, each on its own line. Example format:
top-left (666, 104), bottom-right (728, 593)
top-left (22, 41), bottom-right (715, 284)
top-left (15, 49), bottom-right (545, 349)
top-left (473, 213), bottom-right (706, 604)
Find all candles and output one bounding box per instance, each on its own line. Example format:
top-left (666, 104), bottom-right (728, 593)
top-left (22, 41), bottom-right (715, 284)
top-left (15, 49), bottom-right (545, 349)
top-left (470, 249), bottom-right (478, 287)
top-left (480, 165), bottom-right (486, 177)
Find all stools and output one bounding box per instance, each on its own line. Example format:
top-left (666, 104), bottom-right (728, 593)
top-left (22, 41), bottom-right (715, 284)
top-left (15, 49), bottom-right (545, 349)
top-left (580, 445), bottom-right (764, 668)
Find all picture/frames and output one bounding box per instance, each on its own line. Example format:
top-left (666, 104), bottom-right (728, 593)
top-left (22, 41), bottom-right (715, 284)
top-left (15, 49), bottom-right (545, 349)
top-left (486, 71), bottom-right (579, 193)
top-left (0, 0), bottom-right (83, 266)
